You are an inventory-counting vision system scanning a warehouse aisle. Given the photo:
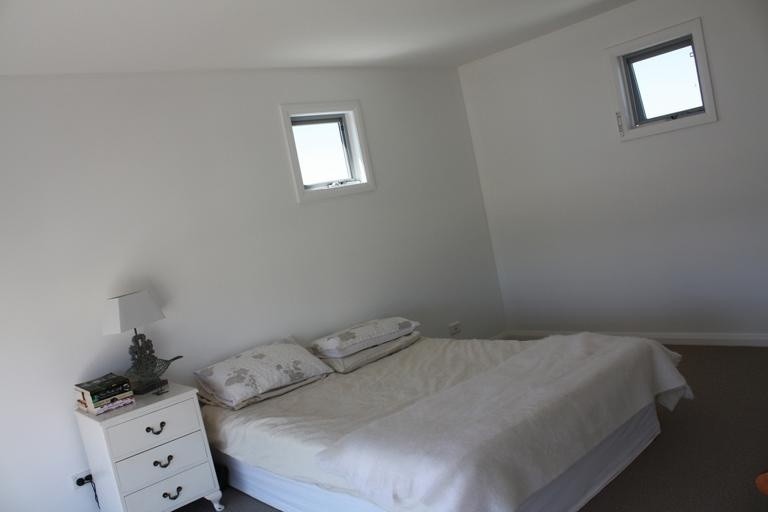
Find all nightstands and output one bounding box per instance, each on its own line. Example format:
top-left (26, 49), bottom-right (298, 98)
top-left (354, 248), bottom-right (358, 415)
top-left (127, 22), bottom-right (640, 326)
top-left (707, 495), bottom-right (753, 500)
top-left (73, 380), bottom-right (226, 511)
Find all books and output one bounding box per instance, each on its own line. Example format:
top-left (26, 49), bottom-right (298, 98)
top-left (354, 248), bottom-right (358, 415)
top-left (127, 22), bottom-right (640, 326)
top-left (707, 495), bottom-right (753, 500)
top-left (72, 372), bottom-right (135, 416)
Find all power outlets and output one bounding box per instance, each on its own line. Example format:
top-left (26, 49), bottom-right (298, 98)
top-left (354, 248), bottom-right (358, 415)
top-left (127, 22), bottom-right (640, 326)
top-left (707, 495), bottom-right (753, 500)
top-left (72, 469), bottom-right (93, 488)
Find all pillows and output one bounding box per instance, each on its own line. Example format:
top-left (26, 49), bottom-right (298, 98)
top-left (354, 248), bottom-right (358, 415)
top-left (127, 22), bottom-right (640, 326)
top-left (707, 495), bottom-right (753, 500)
top-left (195, 374), bottom-right (328, 411)
top-left (191, 334), bottom-right (336, 408)
top-left (316, 330), bottom-right (423, 374)
top-left (306, 314), bottom-right (423, 359)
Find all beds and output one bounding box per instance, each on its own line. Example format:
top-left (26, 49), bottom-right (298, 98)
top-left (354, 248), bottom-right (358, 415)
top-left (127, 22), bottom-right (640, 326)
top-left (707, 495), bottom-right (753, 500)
top-left (199, 333), bottom-right (696, 510)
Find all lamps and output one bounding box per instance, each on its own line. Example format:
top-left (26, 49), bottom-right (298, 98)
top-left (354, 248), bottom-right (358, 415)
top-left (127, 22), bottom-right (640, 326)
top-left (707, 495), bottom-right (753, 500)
top-left (102, 285), bottom-right (168, 396)
